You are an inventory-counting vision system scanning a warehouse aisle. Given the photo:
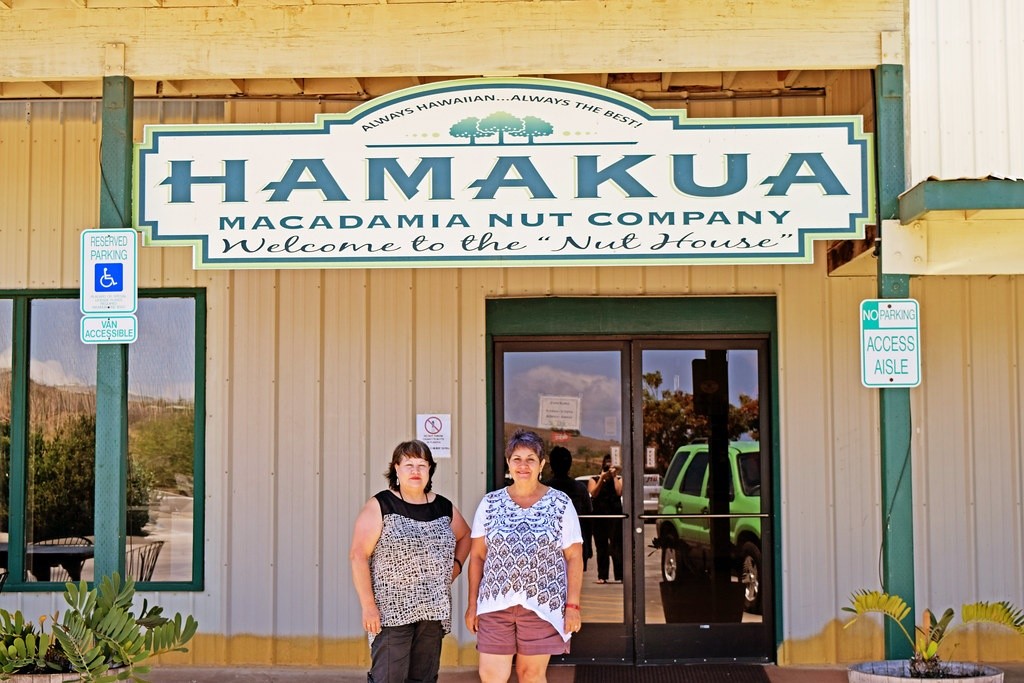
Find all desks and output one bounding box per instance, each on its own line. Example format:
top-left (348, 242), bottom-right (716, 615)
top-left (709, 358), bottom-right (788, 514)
top-left (0, 543), bottom-right (95, 581)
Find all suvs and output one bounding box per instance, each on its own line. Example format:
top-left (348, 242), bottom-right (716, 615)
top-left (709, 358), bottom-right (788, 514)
top-left (656, 441), bottom-right (762, 615)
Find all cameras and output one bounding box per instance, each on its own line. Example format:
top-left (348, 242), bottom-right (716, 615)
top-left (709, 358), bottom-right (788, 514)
top-left (603, 465), bottom-right (609, 472)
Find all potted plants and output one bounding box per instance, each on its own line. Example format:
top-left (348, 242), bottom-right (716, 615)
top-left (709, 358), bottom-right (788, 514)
top-left (0, 570), bottom-right (203, 683)
top-left (839, 588), bottom-right (1023, 683)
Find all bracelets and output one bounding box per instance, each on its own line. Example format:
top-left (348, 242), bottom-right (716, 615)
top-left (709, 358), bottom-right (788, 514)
top-left (566, 604), bottom-right (580, 610)
top-left (454, 559), bottom-right (462, 574)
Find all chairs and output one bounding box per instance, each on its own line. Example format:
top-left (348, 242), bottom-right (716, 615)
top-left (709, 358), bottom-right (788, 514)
top-left (0, 534), bottom-right (166, 585)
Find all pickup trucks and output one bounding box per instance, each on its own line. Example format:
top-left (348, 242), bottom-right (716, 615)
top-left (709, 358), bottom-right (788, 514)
top-left (572, 473), bottom-right (665, 521)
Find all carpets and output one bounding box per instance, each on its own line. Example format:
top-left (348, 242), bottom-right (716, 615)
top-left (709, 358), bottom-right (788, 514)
top-left (574, 664), bottom-right (773, 683)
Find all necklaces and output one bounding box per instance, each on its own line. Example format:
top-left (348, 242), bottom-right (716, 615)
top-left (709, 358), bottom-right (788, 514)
top-left (399, 490), bottom-right (429, 504)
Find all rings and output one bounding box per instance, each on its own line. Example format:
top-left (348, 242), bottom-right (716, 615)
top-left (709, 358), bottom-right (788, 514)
top-left (575, 626), bottom-right (578, 628)
top-left (367, 625), bottom-right (370, 626)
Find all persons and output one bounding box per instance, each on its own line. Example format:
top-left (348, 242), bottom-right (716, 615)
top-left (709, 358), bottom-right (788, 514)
top-left (587, 454), bottom-right (624, 584)
top-left (465, 430), bottom-right (584, 683)
top-left (350, 440), bottom-right (472, 683)
